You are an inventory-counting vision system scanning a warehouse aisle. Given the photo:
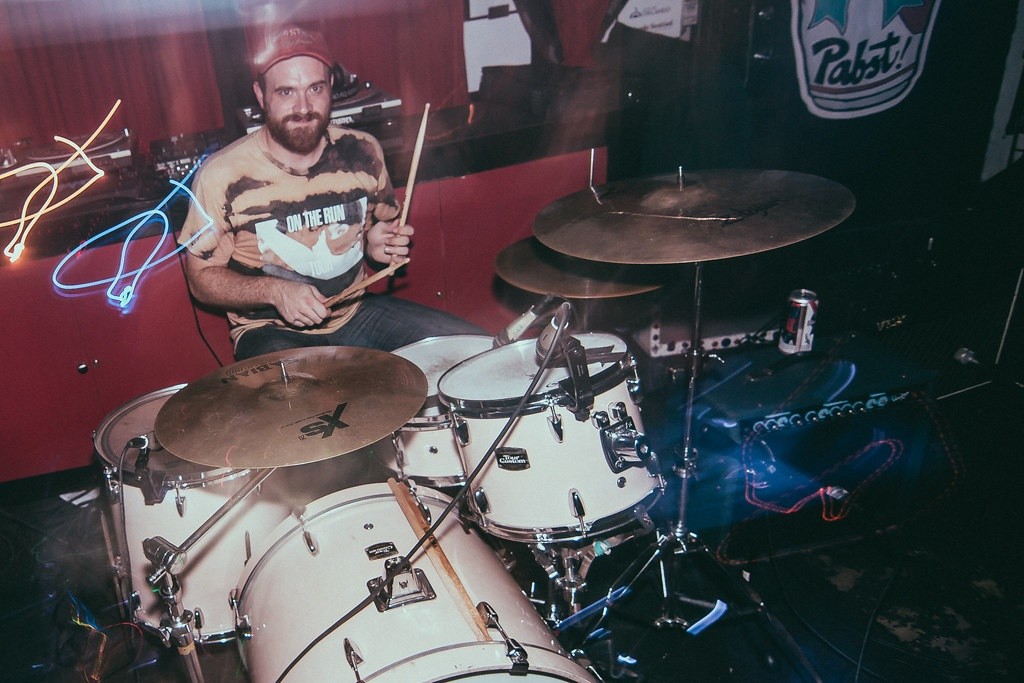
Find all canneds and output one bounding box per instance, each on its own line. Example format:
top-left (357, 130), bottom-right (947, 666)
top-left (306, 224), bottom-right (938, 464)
top-left (778, 288), bottom-right (819, 355)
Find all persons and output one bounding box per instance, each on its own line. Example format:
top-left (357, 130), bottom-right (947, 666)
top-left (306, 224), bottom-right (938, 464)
top-left (177, 25), bottom-right (493, 364)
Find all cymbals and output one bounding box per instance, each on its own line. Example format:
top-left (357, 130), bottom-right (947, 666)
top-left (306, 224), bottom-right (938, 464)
top-left (492, 234), bottom-right (683, 299)
top-left (154, 345), bottom-right (430, 470)
top-left (531, 166), bottom-right (857, 266)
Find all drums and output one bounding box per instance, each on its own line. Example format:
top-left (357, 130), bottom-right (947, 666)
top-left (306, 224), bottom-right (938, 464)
top-left (435, 330), bottom-right (670, 544)
top-left (227, 480), bottom-right (599, 683)
top-left (90, 381), bottom-right (363, 644)
top-left (364, 332), bottom-right (514, 489)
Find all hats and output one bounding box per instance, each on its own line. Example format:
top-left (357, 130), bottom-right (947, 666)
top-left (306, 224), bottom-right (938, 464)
top-left (251, 26), bottom-right (333, 81)
